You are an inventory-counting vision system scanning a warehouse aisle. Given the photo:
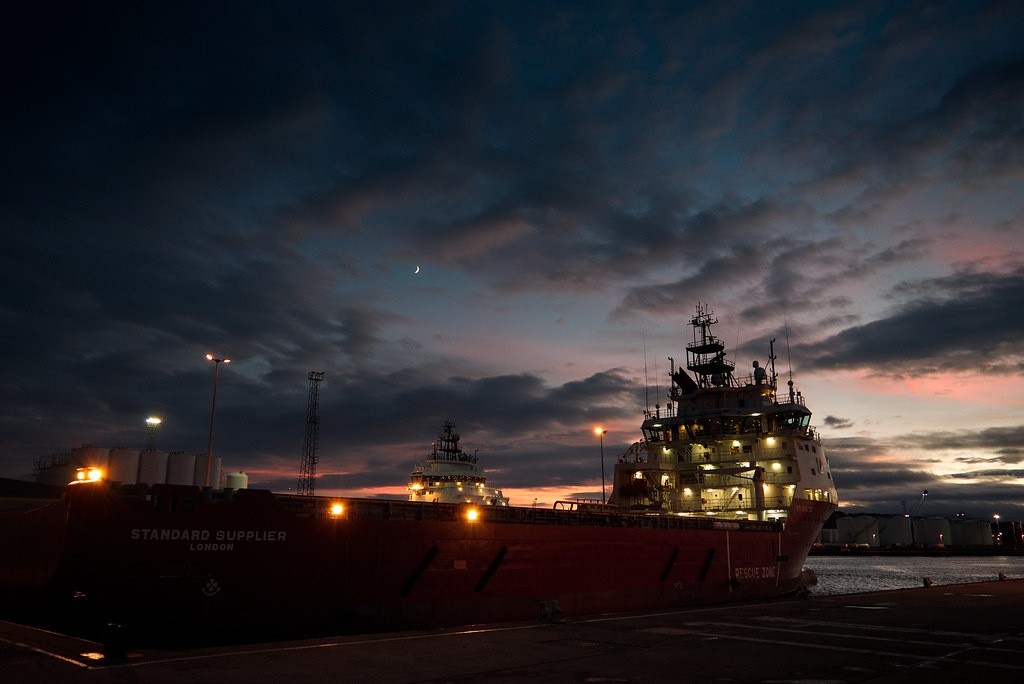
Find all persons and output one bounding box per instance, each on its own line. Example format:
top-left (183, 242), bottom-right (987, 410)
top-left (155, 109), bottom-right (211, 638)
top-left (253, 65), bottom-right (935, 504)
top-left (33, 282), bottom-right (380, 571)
top-left (752, 360), bottom-right (768, 385)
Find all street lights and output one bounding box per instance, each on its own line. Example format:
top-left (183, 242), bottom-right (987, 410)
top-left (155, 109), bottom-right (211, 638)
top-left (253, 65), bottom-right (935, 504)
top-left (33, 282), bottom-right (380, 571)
top-left (205, 353), bottom-right (232, 487)
top-left (594, 429), bottom-right (608, 505)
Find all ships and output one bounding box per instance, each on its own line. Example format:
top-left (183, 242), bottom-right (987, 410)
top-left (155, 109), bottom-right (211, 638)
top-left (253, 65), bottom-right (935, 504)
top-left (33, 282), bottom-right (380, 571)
top-left (0, 303), bottom-right (838, 655)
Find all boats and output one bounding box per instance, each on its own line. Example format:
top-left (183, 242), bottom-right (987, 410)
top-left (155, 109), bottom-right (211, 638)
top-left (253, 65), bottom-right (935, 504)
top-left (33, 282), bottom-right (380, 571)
top-left (406, 419), bottom-right (511, 507)
